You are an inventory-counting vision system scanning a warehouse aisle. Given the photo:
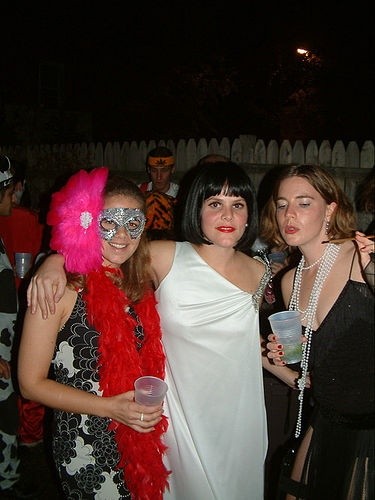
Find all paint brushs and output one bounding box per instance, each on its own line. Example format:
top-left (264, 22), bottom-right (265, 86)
top-left (322, 235), bottom-right (375, 244)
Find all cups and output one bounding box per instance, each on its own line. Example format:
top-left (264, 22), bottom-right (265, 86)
top-left (14, 252), bottom-right (32, 279)
top-left (268, 310), bottom-right (303, 365)
top-left (134, 376), bottom-right (168, 408)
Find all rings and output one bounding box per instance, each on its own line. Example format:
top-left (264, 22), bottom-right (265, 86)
top-left (140, 412), bottom-right (144, 422)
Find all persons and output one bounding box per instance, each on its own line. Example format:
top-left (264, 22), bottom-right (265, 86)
top-left (0, 158), bottom-right (47, 293)
top-left (0, 158), bottom-right (20, 500)
top-left (26, 160), bottom-right (375, 499)
top-left (140, 146), bottom-right (179, 199)
top-left (259, 163), bottom-right (375, 500)
top-left (17, 167), bottom-right (171, 500)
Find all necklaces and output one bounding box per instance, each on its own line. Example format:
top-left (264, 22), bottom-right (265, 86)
top-left (287, 242), bottom-right (339, 438)
